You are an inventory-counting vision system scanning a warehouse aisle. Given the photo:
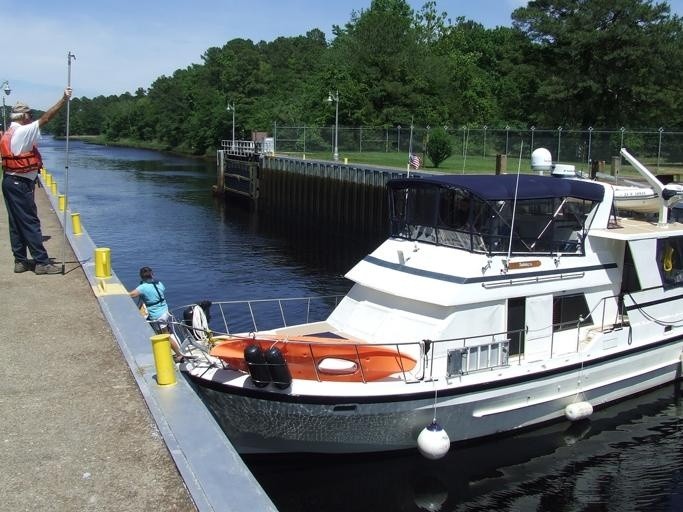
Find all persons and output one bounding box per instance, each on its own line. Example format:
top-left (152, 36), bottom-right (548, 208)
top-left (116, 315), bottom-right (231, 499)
top-left (0, 85), bottom-right (73, 276)
top-left (127, 265), bottom-right (186, 364)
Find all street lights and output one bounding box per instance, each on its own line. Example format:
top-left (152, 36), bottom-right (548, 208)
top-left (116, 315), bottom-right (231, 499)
top-left (225, 102), bottom-right (235, 146)
top-left (0, 80), bottom-right (11, 95)
top-left (326, 90), bottom-right (338, 153)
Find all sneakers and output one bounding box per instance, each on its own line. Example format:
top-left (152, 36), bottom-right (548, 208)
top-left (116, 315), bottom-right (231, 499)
top-left (15, 262), bottom-right (62, 274)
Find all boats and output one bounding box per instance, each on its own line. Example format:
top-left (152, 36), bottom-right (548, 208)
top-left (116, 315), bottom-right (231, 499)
top-left (153, 149), bottom-right (681, 456)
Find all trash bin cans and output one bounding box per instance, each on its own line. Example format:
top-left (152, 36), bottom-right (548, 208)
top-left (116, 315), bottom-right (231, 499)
top-left (592, 161), bottom-right (605, 177)
top-left (611, 157), bottom-right (621, 176)
top-left (496, 155), bottom-right (507, 175)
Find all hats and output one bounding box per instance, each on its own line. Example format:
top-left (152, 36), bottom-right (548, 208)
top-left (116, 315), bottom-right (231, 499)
top-left (11, 104), bottom-right (31, 113)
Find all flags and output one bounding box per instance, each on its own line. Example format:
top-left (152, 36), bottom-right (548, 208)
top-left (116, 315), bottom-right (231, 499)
top-left (407, 154), bottom-right (421, 170)
